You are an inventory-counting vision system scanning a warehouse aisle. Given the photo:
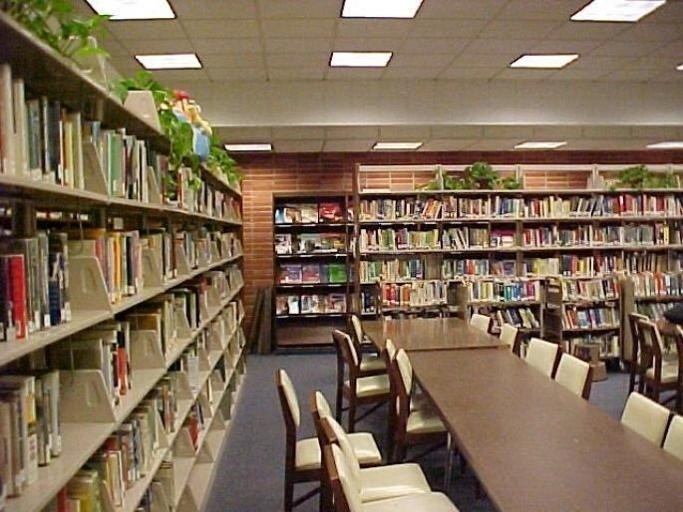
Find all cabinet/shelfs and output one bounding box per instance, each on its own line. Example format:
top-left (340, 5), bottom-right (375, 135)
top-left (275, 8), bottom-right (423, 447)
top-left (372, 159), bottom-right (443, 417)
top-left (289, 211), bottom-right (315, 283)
top-left (1, 13), bottom-right (249, 512)
top-left (270, 161), bottom-right (682, 354)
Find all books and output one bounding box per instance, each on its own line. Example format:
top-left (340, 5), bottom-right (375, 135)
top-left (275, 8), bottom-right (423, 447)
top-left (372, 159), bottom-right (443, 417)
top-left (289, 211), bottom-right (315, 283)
top-left (0, 64), bottom-right (247, 512)
top-left (274, 194), bottom-right (683, 354)
